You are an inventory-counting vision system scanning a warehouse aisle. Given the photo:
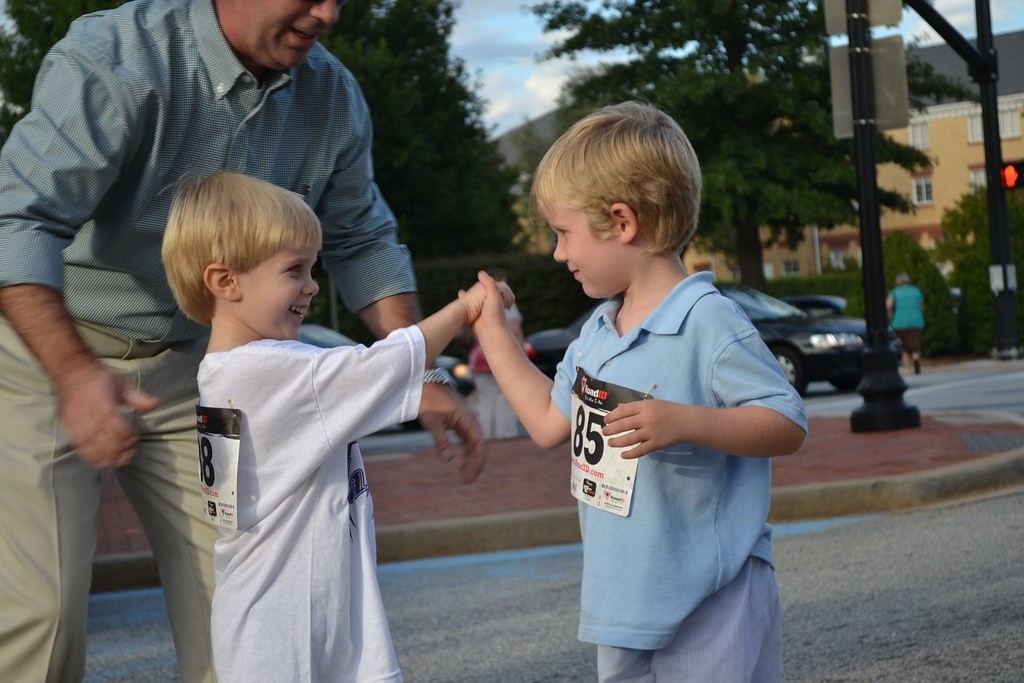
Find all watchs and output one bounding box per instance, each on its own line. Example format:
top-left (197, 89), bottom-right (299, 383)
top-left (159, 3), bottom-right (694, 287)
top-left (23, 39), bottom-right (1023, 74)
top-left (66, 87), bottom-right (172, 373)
top-left (423, 366), bottom-right (456, 389)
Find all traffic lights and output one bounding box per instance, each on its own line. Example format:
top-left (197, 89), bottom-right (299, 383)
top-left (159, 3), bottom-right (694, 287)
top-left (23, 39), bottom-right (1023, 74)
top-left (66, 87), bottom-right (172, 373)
top-left (1003, 161), bottom-right (1024, 190)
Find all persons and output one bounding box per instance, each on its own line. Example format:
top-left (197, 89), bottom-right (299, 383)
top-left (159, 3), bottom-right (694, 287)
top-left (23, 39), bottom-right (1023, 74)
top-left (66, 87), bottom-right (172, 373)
top-left (886, 272), bottom-right (925, 376)
top-left (161, 168), bottom-right (517, 683)
top-left (458, 99), bottom-right (808, 683)
top-left (0, 1), bottom-right (486, 682)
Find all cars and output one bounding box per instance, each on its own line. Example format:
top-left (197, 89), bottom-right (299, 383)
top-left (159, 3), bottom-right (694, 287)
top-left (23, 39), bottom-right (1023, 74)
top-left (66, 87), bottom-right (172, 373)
top-left (297, 322), bottom-right (476, 431)
top-left (520, 282), bottom-right (902, 398)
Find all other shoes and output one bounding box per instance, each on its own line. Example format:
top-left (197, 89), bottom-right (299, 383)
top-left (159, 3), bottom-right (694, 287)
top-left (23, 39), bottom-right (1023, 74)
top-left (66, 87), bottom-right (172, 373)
top-left (914, 360), bottom-right (920, 373)
